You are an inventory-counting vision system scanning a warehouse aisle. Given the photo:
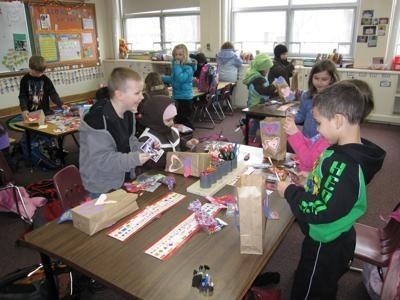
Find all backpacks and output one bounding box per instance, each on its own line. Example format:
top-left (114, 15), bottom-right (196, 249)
top-left (197, 62), bottom-right (220, 95)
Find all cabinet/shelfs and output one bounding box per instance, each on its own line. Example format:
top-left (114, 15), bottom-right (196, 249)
top-left (100, 58), bottom-right (400, 123)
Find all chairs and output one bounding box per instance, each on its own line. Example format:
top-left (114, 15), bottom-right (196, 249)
top-left (53, 162), bottom-right (86, 210)
top-left (347, 205), bottom-right (400, 299)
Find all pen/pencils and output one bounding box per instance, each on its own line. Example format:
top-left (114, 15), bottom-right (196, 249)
top-left (268, 156), bottom-right (280, 181)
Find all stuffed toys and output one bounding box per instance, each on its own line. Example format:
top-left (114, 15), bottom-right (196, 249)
top-left (120, 38), bottom-right (129, 58)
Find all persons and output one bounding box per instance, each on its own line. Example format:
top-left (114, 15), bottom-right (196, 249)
top-left (288, 59), bottom-right (340, 139)
top-left (276, 83), bottom-right (385, 300)
top-left (18, 55), bottom-right (68, 166)
top-left (136, 94), bottom-right (200, 176)
top-left (284, 79), bottom-right (375, 173)
top-left (239, 53), bottom-right (274, 147)
top-left (268, 45), bottom-right (296, 88)
top-left (161, 42), bottom-right (243, 132)
top-left (0, 120), bottom-right (19, 174)
top-left (134, 70), bottom-right (169, 136)
top-left (79, 67), bottom-right (151, 201)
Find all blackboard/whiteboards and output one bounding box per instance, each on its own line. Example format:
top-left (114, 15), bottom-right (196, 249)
top-left (0, 0), bottom-right (37, 78)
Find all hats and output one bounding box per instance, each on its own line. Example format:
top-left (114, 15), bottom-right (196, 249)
top-left (253, 53), bottom-right (274, 71)
top-left (163, 103), bottom-right (178, 120)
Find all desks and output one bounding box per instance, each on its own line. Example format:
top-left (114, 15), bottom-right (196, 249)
top-left (15, 141), bottom-right (312, 300)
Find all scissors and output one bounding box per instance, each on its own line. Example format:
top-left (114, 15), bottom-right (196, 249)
top-left (218, 142), bottom-right (241, 161)
